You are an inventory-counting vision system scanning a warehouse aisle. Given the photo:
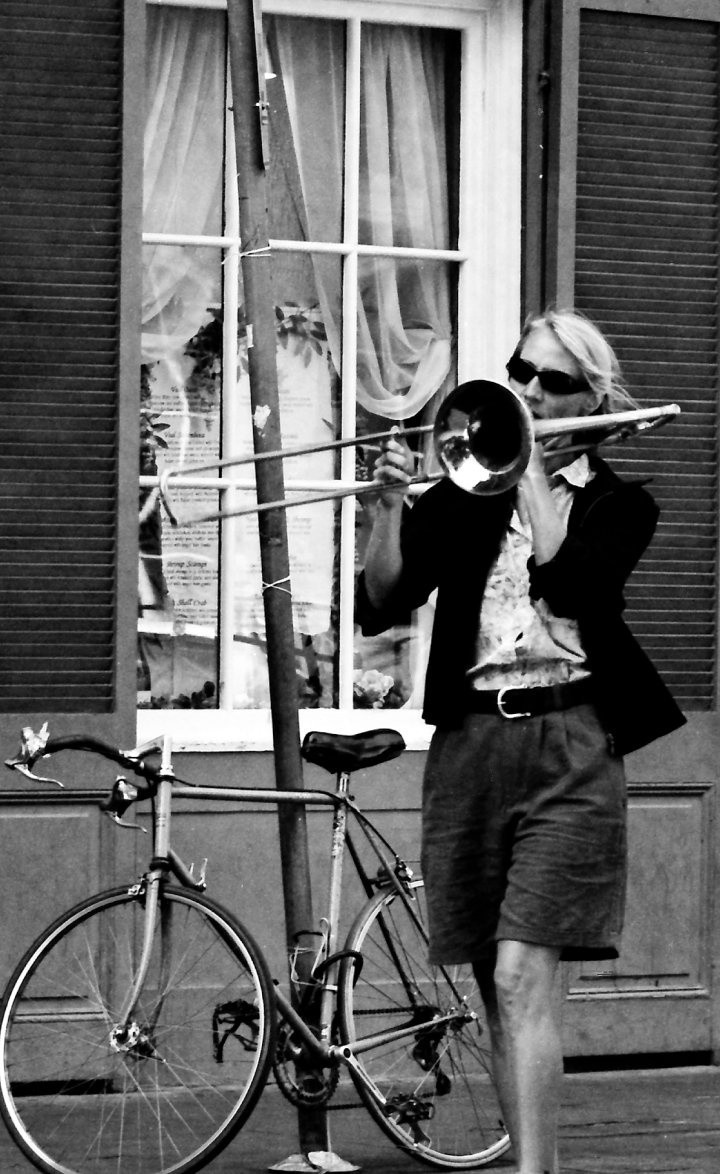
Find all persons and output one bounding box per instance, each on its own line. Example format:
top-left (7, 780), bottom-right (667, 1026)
top-left (352, 307), bottom-right (689, 1174)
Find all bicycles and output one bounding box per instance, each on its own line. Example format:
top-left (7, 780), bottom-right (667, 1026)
top-left (1, 722), bottom-right (518, 1174)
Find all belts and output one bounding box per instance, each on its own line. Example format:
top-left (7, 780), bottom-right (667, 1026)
top-left (472, 677), bottom-right (592, 720)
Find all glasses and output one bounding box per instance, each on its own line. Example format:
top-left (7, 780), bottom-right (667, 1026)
top-left (505, 353), bottom-right (591, 396)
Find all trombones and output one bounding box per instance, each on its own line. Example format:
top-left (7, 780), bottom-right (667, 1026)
top-left (159, 378), bottom-right (681, 530)
